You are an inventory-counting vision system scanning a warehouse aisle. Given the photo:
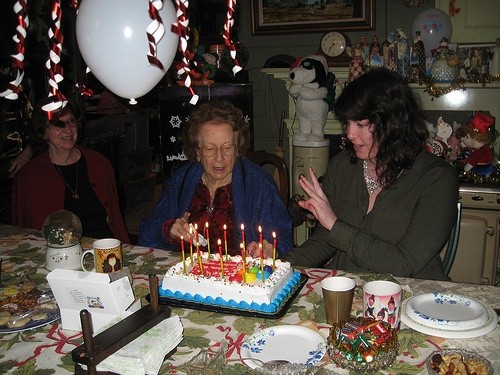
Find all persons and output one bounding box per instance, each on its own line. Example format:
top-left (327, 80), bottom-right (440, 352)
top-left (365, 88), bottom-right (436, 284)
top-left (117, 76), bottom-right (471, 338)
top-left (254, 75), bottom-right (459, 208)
top-left (0, 77), bottom-right (34, 225)
top-left (10, 103), bottom-right (131, 244)
top-left (136, 98), bottom-right (294, 260)
top-left (348, 30), bottom-right (500, 83)
top-left (279, 67), bottom-right (460, 281)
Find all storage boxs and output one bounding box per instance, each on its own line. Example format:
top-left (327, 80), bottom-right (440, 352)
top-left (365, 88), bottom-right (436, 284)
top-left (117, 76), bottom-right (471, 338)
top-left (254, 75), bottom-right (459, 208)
top-left (47, 269), bottom-right (141, 333)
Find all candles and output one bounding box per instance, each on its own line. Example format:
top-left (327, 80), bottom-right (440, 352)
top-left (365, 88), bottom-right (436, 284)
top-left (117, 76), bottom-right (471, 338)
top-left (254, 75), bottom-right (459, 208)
top-left (179, 222), bottom-right (276, 282)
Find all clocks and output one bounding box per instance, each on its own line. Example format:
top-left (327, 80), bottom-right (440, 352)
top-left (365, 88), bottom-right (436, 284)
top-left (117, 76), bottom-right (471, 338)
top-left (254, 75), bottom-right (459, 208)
top-left (320, 30), bottom-right (354, 66)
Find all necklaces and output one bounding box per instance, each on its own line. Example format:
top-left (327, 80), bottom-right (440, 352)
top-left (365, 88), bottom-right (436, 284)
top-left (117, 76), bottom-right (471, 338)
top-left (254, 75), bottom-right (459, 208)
top-left (51, 151), bottom-right (80, 200)
top-left (203, 174), bottom-right (232, 213)
top-left (363, 160), bottom-right (403, 193)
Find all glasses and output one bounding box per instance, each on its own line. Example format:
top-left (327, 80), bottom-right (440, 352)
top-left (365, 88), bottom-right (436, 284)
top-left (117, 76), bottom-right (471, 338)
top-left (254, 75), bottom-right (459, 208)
top-left (196, 141), bottom-right (240, 157)
top-left (47, 116), bottom-right (78, 131)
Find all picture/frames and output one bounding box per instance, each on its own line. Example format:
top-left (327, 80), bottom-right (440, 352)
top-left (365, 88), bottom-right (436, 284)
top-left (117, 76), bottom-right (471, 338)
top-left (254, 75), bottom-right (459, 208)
top-left (454, 42), bottom-right (497, 83)
top-left (250, 1), bottom-right (376, 36)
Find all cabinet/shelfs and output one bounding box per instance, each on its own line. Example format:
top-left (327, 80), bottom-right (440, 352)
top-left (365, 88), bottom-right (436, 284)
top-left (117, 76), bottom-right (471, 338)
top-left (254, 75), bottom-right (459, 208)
top-left (260, 64), bottom-right (500, 285)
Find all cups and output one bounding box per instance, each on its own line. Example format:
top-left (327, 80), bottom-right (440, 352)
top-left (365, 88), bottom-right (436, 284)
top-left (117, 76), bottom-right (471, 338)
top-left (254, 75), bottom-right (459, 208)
top-left (320, 276), bottom-right (356, 325)
top-left (81, 238), bottom-right (122, 274)
top-left (362, 281), bottom-right (401, 331)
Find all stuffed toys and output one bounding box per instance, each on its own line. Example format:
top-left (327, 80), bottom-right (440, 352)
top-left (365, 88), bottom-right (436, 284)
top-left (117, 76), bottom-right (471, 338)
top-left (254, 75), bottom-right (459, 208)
top-left (426, 111), bottom-right (495, 178)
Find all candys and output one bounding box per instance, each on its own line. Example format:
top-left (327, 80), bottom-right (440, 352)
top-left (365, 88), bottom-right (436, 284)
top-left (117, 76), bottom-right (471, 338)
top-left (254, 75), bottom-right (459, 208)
top-left (332, 319), bottom-right (390, 363)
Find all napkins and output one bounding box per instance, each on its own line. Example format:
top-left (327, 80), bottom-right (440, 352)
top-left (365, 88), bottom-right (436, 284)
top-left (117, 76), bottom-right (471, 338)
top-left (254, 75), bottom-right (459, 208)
top-left (82, 316), bottom-right (183, 373)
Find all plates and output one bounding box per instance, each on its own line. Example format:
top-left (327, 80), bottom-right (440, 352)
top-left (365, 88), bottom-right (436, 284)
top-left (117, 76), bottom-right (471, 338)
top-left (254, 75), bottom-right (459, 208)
top-left (240, 325), bottom-right (327, 371)
top-left (425, 349), bottom-right (494, 375)
top-left (327, 345), bottom-right (398, 371)
top-left (401, 291), bottom-right (498, 338)
top-left (0, 288), bottom-right (60, 335)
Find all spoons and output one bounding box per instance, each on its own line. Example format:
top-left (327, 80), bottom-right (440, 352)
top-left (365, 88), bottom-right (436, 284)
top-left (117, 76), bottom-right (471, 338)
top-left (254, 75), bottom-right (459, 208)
top-left (226, 358), bottom-right (289, 369)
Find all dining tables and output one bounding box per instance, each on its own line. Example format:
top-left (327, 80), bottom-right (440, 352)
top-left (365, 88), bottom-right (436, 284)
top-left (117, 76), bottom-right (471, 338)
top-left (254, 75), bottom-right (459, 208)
top-left (0, 219), bottom-right (500, 375)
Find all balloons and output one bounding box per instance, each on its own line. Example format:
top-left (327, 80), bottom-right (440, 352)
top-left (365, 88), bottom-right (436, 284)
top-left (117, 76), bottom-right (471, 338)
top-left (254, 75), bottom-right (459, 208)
top-left (410, 8), bottom-right (452, 57)
top-left (75, 0), bottom-right (179, 104)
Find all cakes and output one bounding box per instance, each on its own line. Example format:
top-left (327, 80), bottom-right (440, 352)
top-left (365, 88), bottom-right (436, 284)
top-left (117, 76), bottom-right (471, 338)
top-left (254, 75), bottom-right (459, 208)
top-left (157, 249), bottom-right (302, 313)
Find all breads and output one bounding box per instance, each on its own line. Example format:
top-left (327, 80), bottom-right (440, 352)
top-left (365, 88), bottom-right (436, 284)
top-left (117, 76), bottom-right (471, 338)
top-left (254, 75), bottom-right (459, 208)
top-left (0, 281), bottom-right (57, 328)
